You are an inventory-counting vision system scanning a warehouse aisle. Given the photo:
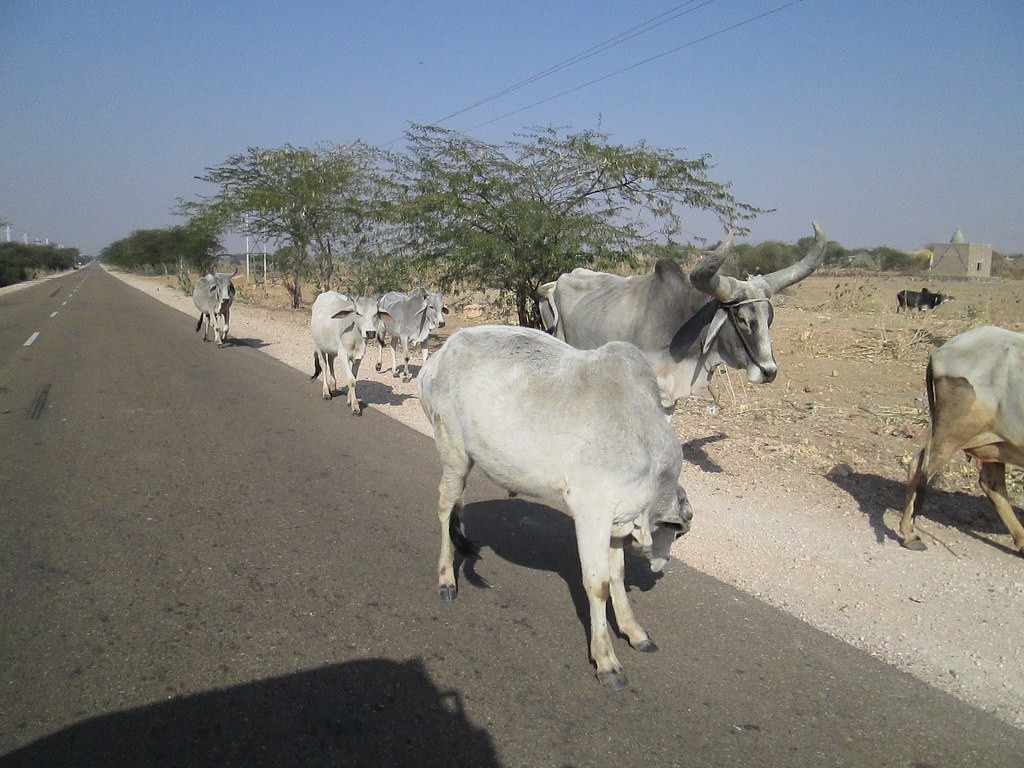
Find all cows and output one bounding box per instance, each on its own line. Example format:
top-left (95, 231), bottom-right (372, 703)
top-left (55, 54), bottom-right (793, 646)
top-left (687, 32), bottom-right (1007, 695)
top-left (374, 286), bottom-right (450, 384)
top-left (415, 324), bottom-right (694, 692)
top-left (898, 324), bottom-right (1024, 559)
top-left (896, 287), bottom-right (957, 320)
top-left (309, 289), bottom-right (394, 417)
top-left (191, 265), bottom-right (240, 350)
top-left (536, 219), bottom-right (829, 425)
